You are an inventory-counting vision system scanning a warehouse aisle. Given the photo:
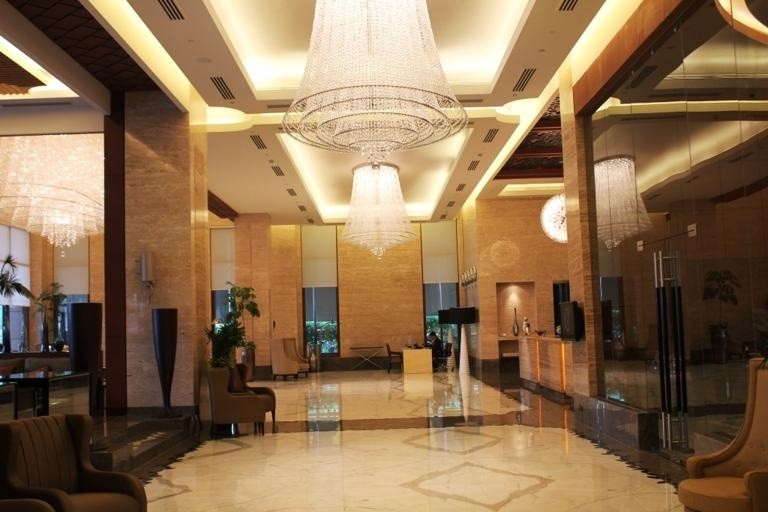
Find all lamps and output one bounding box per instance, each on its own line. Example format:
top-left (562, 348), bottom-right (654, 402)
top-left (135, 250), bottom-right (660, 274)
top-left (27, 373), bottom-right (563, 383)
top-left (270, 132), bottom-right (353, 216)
top-left (593, 123), bottom-right (653, 253)
top-left (140, 251), bottom-right (155, 304)
top-left (283, 1), bottom-right (468, 260)
top-left (540, 193), bottom-right (567, 243)
top-left (0, 133), bottom-right (104, 259)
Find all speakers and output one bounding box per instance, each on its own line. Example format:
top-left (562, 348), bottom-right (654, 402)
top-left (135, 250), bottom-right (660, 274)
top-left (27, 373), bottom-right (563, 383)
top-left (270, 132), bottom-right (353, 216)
top-left (437, 307), bottom-right (477, 325)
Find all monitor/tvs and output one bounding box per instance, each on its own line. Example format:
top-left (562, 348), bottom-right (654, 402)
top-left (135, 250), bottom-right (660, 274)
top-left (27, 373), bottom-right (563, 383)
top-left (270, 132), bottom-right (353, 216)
top-left (559, 301), bottom-right (585, 342)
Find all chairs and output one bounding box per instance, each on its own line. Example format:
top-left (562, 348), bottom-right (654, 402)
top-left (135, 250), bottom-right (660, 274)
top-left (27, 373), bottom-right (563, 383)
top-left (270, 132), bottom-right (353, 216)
top-left (206, 367), bottom-right (276, 436)
top-left (271, 337), bottom-right (310, 381)
top-left (437, 343), bottom-right (452, 372)
top-left (386, 342), bottom-right (403, 373)
top-left (679, 358), bottom-right (768, 511)
top-left (0, 414), bottom-right (147, 512)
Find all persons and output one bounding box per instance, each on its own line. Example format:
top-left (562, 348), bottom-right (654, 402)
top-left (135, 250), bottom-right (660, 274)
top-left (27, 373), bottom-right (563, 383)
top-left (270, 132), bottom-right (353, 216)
top-left (427, 331), bottom-right (442, 357)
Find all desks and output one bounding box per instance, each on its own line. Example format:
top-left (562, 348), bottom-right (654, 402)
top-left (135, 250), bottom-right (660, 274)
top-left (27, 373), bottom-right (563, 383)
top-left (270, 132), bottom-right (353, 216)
top-left (402, 347), bottom-right (433, 374)
top-left (350, 347), bottom-right (384, 370)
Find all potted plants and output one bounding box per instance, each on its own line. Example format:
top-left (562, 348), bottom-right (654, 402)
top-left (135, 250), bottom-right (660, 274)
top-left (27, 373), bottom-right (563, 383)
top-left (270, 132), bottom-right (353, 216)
top-left (702, 269), bottom-right (741, 362)
top-left (225, 279), bottom-right (260, 382)
